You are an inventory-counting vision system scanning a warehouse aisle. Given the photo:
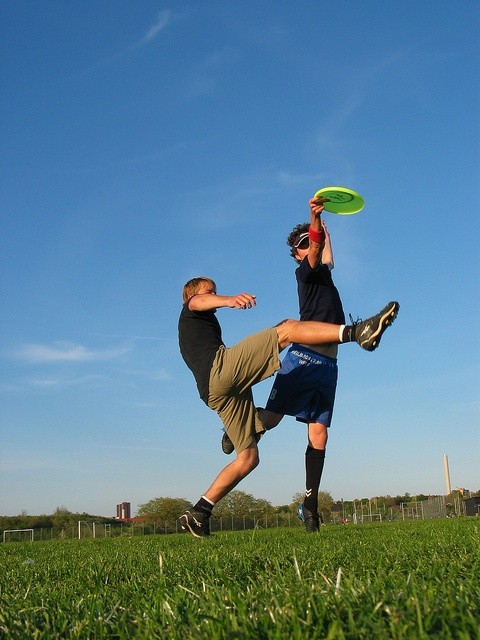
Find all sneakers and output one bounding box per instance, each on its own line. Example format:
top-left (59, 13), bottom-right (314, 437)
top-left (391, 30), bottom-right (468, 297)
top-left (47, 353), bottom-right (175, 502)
top-left (222, 430), bottom-right (234, 454)
top-left (354, 301), bottom-right (400, 351)
top-left (297, 503), bottom-right (320, 534)
top-left (179, 509), bottom-right (210, 538)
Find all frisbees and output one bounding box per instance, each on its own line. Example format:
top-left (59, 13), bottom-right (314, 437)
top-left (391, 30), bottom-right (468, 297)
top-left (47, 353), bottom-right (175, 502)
top-left (314, 187), bottom-right (365, 215)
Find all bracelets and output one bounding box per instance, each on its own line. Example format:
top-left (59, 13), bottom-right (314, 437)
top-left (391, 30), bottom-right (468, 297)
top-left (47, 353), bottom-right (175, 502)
top-left (309, 225), bottom-right (326, 243)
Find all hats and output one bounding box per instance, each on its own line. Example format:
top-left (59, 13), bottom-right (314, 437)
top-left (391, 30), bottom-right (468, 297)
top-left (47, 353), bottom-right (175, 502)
top-left (293, 232), bottom-right (309, 247)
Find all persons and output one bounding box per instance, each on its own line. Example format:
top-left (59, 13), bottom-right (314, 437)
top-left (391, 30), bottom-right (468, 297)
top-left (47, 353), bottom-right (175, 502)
top-left (178, 274), bottom-right (400, 539)
top-left (220, 196), bottom-right (345, 533)
